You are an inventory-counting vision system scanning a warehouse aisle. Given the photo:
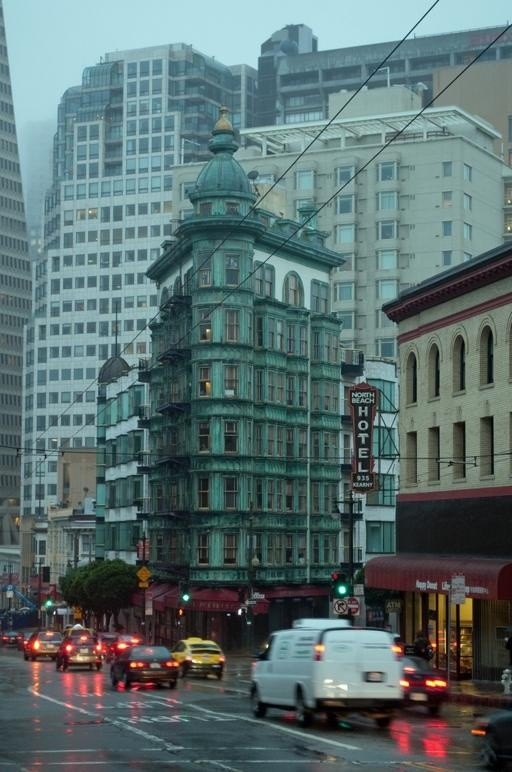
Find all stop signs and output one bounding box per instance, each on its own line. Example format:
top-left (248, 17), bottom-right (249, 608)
top-left (344, 597), bottom-right (360, 617)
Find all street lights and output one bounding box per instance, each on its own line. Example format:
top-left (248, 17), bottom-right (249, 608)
top-left (329, 489), bottom-right (363, 620)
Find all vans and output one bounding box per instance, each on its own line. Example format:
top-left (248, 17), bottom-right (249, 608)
top-left (248, 620), bottom-right (404, 728)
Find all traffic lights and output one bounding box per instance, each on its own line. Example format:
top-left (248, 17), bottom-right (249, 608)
top-left (176, 607), bottom-right (186, 618)
top-left (333, 572), bottom-right (347, 599)
top-left (46, 596), bottom-right (51, 607)
top-left (179, 581), bottom-right (190, 604)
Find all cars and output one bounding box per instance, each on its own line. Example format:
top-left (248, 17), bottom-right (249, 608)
top-left (96, 631), bottom-right (122, 648)
top-left (169, 637), bottom-right (226, 679)
top-left (24, 630), bottom-right (64, 662)
top-left (55, 635), bottom-right (104, 672)
top-left (2, 630), bottom-right (35, 651)
top-left (470, 705), bottom-right (512, 767)
top-left (109, 645), bottom-right (181, 690)
top-left (106, 635), bottom-right (145, 663)
top-left (388, 655), bottom-right (449, 718)
top-left (63, 624), bottom-right (93, 637)
